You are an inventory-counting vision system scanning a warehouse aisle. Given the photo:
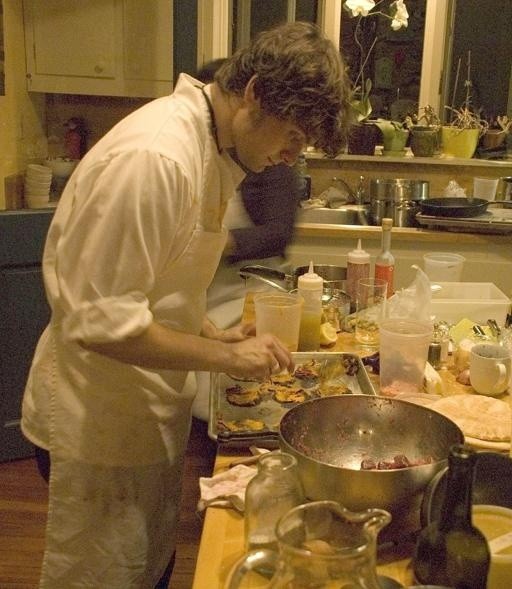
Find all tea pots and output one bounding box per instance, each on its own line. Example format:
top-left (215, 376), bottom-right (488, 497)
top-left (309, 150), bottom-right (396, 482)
top-left (221, 501), bottom-right (393, 589)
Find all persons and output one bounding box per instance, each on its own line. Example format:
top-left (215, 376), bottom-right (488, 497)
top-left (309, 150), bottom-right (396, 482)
top-left (17, 20), bottom-right (352, 588)
top-left (196, 57), bottom-right (301, 422)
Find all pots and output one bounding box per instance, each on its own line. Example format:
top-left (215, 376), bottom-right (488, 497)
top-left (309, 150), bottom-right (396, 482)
top-left (369, 177), bottom-right (429, 228)
top-left (420, 198), bottom-right (512, 216)
top-left (216, 394), bottom-right (466, 524)
top-left (235, 264), bottom-right (351, 320)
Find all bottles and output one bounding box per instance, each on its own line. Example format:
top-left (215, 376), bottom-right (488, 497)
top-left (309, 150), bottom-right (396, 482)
top-left (412, 443), bottom-right (492, 589)
top-left (346, 238), bottom-right (371, 312)
top-left (296, 260), bottom-right (323, 351)
top-left (374, 216), bottom-right (395, 301)
top-left (244, 452), bottom-right (306, 552)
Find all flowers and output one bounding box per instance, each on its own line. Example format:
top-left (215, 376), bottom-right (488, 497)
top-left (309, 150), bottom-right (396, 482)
top-left (343, 0), bottom-right (410, 126)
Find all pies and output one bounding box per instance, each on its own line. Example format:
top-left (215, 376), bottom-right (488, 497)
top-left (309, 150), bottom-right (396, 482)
top-left (429, 394), bottom-right (512, 442)
top-left (463, 437), bottom-right (511, 453)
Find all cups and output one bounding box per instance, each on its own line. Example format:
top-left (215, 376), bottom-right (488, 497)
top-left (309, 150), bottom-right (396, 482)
top-left (378, 321), bottom-right (434, 397)
top-left (356, 277), bottom-right (388, 344)
top-left (469, 343), bottom-right (512, 396)
top-left (422, 252), bottom-right (466, 282)
top-left (474, 176), bottom-right (512, 208)
top-left (254, 294), bottom-right (302, 351)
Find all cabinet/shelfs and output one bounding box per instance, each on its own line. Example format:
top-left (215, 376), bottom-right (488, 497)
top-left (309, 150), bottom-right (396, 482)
top-left (22, 0), bottom-right (174, 98)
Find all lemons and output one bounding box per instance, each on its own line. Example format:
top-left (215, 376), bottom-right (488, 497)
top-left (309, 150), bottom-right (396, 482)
top-left (320, 322), bottom-right (337, 345)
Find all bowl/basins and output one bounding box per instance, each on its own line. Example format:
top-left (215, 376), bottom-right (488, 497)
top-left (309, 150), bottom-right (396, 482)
top-left (43, 156), bottom-right (80, 179)
top-left (22, 163), bottom-right (52, 209)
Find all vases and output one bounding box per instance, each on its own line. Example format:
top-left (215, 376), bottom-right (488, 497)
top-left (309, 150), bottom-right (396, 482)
top-left (347, 117), bottom-right (505, 159)
